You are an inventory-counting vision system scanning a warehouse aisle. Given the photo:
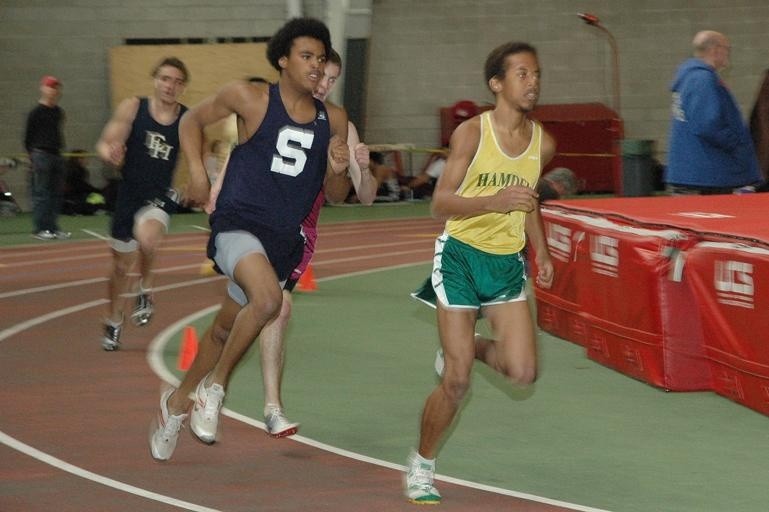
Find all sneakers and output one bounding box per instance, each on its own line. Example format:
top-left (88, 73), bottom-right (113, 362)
top-left (33, 230), bottom-right (70, 241)
top-left (190, 372), bottom-right (226, 446)
top-left (265, 408), bottom-right (298, 438)
top-left (406, 453), bottom-right (441, 505)
top-left (132, 280), bottom-right (156, 325)
top-left (150, 388), bottom-right (187, 461)
top-left (101, 310), bottom-right (125, 353)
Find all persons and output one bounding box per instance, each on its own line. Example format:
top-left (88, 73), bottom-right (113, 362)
top-left (55, 148), bottom-right (117, 216)
top-left (24, 75), bottom-right (69, 240)
top-left (150, 16), bottom-right (349, 461)
top-left (94, 57), bottom-right (190, 350)
top-left (402, 40), bottom-right (555, 504)
top-left (205, 48), bottom-right (378, 438)
top-left (205, 141), bottom-right (452, 218)
top-left (663, 30), bottom-right (760, 194)
top-left (535, 168), bottom-right (577, 204)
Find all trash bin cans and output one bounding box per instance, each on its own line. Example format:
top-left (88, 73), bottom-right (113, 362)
top-left (623, 139), bottom-right (655, 197)
